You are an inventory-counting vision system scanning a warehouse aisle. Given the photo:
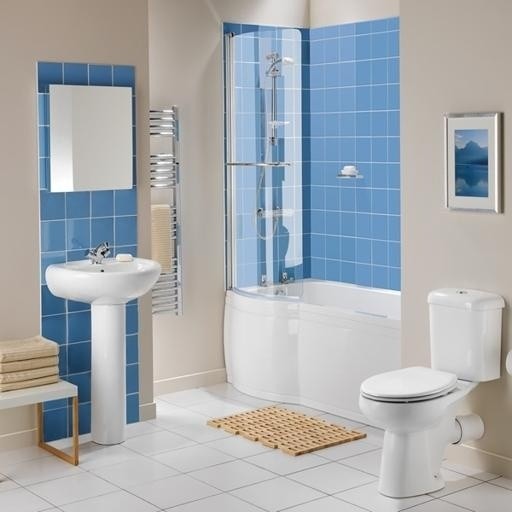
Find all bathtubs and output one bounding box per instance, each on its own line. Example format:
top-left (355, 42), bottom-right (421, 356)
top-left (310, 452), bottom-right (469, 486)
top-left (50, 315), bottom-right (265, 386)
top-left (223, 277), bottom-right (401, 430)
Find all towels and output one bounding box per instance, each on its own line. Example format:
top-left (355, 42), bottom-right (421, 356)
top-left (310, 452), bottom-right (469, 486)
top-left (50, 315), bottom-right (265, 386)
top-left (0, 335), bottom-right (59, 392)
top-left (150, 203), bottom-right (173, 275)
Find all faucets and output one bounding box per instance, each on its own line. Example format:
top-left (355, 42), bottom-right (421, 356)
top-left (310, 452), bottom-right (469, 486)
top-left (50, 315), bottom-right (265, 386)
top-left (282, 272), bottom-right (295, 284)
top-left (85, 240), bottom-right (112, 265)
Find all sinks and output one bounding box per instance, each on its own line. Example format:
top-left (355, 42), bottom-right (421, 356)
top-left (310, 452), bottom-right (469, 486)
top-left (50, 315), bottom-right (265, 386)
top-left (46, 256), bottom-right (162, 303)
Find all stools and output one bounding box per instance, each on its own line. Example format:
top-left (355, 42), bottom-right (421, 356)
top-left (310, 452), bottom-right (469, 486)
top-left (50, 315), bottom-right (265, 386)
top-left (0, 379), bottom-right (80, 467)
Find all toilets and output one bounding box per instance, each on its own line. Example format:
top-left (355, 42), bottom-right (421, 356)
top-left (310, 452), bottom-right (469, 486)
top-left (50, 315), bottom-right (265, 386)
top-left (360, 286), bottom-right (505, 498)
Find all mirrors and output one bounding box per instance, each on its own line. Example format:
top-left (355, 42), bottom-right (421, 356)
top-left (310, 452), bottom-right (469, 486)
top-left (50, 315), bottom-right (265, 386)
top-left (48, 83), bottom-right (134, 194)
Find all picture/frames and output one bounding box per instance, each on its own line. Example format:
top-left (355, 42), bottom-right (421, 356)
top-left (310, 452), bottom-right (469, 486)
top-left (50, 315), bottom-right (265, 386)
top-left (442, 111), bottom-right (503, 215)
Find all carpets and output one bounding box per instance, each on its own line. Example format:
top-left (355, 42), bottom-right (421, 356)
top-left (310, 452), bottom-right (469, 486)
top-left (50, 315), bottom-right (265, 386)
top-left (207, 404), bottom-right (368, 458)
